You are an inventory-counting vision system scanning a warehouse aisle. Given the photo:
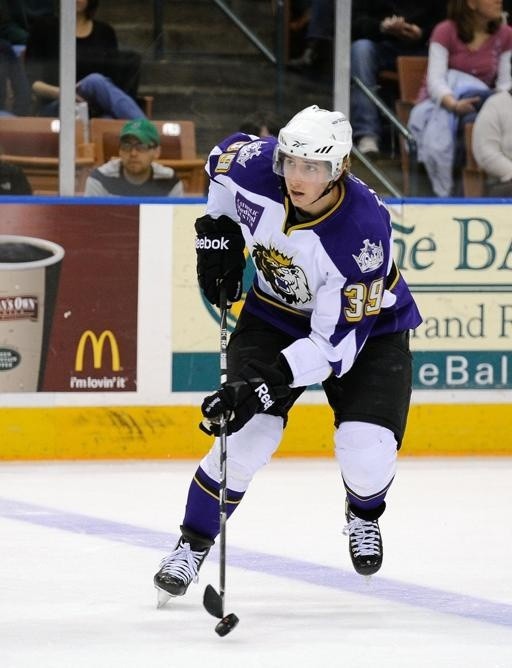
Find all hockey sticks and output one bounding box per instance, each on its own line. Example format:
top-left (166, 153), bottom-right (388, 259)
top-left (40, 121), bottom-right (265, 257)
top-left (203, 282), bottom-right (227, 618)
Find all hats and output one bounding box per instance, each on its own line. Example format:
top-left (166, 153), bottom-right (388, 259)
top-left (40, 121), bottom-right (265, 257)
top-left (120, 119), bottom-right (160, 147)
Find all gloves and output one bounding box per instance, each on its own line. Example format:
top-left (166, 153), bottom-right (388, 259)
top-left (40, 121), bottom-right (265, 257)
top-left (194, 214), bottom-right (246, 308)
top-left (199, 349), bottom-right (294, 437)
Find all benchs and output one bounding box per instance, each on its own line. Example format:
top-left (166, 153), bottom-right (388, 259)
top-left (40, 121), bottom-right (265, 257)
top-left (276, 2), bottom-right (510, 196)
top-left (0, 43), bottom-right (206, 195)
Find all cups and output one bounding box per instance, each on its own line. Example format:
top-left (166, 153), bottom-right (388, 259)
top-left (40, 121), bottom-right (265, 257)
top-left (0, 234), bottom-right (68, 394)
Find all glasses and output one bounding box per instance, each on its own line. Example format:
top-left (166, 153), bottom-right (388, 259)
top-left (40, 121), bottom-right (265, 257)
top-left (121, 142), bottom-right (156, 152)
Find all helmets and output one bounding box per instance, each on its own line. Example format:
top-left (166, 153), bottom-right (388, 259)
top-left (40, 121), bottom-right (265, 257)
top-left (272, 105), bottom-right (353, 180)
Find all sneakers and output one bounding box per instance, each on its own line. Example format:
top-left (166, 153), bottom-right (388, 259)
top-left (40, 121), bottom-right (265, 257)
top-left (358, 137), bottom-right (380, 156)
top-left (154, 526), bottom-right (215, 595)
top-left (346, 499), bottom-right (383, 575)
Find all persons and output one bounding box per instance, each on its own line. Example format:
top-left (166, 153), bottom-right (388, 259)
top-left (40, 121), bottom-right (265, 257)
top-left (23, 1), bottom-right (148, 120)
top-left (1, 1), bottom-right (46, 119)
top-left (154, 104), bottom-right (424, 597)
top-left (84, 118), bottom-right (184, 196)
top-left (274, 0), bottom-right (512, 197)
